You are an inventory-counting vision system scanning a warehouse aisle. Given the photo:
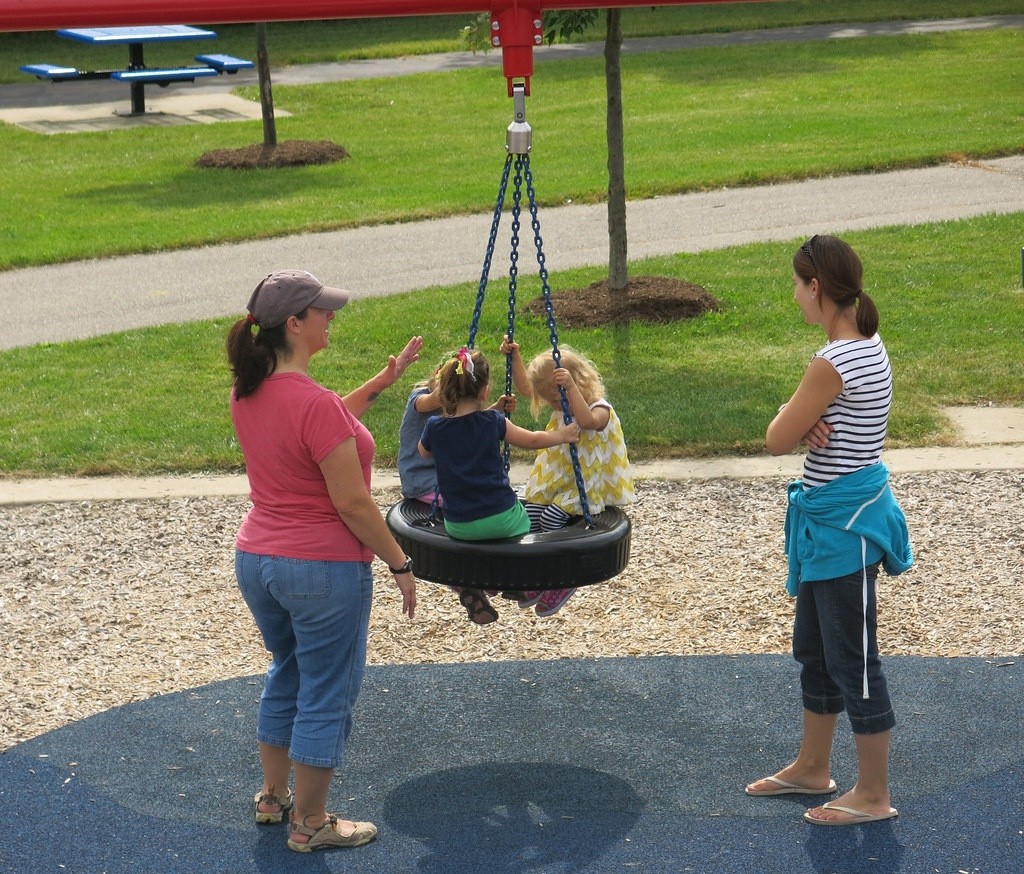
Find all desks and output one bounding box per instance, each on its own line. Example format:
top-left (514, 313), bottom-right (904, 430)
top-left (50, 23), bottom-right (220, 118)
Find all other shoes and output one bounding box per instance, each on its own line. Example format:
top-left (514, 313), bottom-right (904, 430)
top-left (483, 588), bottom-right (498, 597)
top-left (459, 589), bottom-right (499, 624)
top-left (517, 590), bottom-right (544, 609)
top-left (534, 588), bottom-right (577, 616)
top-left (500, 592), bottom-right (522, 601)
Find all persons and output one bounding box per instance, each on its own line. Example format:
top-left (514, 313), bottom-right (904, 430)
top-left (397, 368), bottom-right (517, 508)
top-left (500, 334), bottom-right (638, 616)
top-left (227, 269), bottom-right (423, 853)
top-left (744, 234), bottom-right (913, 825)
top-left (417, 344), bottom-right (581, 624)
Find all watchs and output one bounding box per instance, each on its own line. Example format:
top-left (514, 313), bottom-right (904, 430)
top-left (389, 555), bottom-right (414, 574)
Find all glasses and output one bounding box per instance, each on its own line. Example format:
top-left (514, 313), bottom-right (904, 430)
top-left (800, 234), bottom-right (820, 288)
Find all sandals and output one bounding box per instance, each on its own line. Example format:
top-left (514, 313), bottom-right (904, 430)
top-left (254, 786), bottom-right (293, 824)
top-left (288, 809), bottom-right (378, 853)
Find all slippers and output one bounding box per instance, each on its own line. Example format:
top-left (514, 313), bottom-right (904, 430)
top-left (803, 800), bottom-right (901, 825)
top-left (744, 775), bottom-right (837, 796)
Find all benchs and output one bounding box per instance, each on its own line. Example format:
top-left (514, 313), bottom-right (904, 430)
top-left (112, 66), bottom-right (219, 83)
top-left (196, 54), bottom-right (256, 72)
top-left (19, 62), bottom-right (77, 81)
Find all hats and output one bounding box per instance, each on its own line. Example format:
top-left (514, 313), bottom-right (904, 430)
top-left (247, 272), bottom-right (349, 329)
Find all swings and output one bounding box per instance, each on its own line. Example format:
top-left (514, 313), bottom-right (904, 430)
top-left (385, 152), bottom-right (632, 593)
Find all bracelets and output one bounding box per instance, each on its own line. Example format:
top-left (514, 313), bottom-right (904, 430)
top-left (778, 404), bottom-right (787, 411)
